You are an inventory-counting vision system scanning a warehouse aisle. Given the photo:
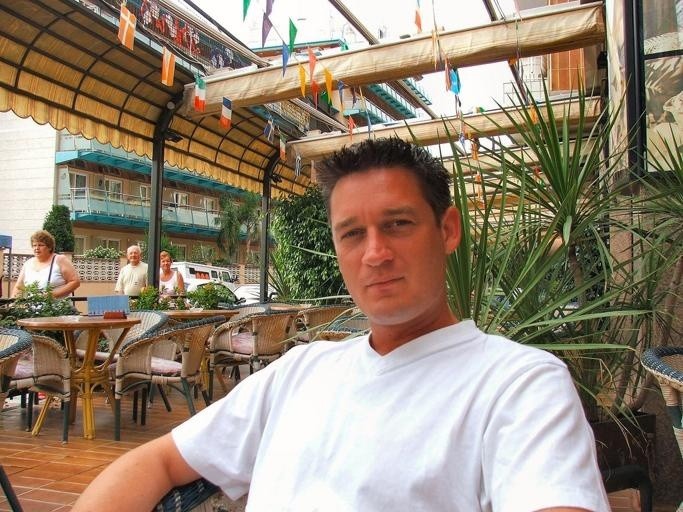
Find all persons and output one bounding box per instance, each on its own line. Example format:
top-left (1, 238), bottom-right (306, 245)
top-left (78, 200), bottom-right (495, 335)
top-left (12, 230), bottom-right (80, 399)
top-left (70, 137), bottom-right (613, 512)
top-left (159, 251), bottom-right (193, 310)
top-left (115, 246), bottom-right (148, 299)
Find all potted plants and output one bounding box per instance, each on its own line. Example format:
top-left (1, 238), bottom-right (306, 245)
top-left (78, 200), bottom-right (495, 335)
top-left (403, 63), bottom-right (683, 489)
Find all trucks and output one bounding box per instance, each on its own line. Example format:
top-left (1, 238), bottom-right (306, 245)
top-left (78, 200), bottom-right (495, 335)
top-left (163, 259), bottom-right (235, 297)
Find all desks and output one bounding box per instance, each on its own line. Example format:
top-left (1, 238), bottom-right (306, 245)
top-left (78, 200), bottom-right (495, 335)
top-left (17, 314), bottom-right (143, 439)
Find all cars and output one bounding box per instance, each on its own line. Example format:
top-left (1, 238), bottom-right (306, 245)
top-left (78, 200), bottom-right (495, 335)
top-left (476, 287), bottom-right (587, 331)
top-left (180, 279), bottom-right (248, 313)
top-left (222, 281), bottom-right (279, 310)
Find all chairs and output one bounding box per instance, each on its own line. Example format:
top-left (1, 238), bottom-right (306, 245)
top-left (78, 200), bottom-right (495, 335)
top-left (71, 310), bottom-right (168, 363)
top-left (114, 314), bottom-right (227, 442)
top-left (641, 346), bottom-right (683, 512)
top-left (0, 326), bottom-right (73, 443)
top-left (161, 301), bottom-right (369, 402)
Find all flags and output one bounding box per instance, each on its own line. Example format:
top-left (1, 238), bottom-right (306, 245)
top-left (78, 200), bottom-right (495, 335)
top-left (220, 97), bottom-right (231, 129)
top-left (264, 117), bottom-right (275, 143)
top-left (161, 47), bottom-right (175, 86)
top-left (279, 138), bottom-right (287, 160)
top-left (311, 160), bottom-right (323, 183)
top-left (295, 152), bottom-right (302, 178)
top-left (195, 75), bottom-right (206, 112)
top-left (118, 5), bottom-right (136, 50)
